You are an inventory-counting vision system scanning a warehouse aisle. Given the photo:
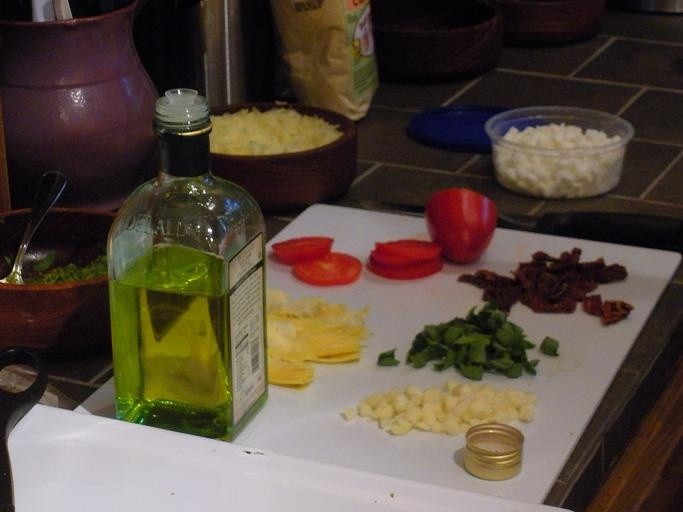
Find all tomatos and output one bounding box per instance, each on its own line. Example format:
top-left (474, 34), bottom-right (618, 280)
top-left (271, 187), bottom-right (497, 286)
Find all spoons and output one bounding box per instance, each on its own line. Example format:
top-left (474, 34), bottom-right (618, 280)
top-left (1, 170), bottom-right (67, 283)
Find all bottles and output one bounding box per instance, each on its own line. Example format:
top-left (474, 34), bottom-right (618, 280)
top-left (0, 2), bottom-right (160, 213)
top-left (102, 86), bottom-right (271, 439)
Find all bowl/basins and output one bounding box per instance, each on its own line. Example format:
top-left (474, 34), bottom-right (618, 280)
top-left (0, 206), bottom-right (117, 358)
top-left (478, 105), bottom-right (636, 198)
top-left (204, 103), bottom-right (362, 213)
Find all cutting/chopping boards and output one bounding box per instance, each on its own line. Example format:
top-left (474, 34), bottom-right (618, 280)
top-left (73, 202), bottom-right (682, 500)
